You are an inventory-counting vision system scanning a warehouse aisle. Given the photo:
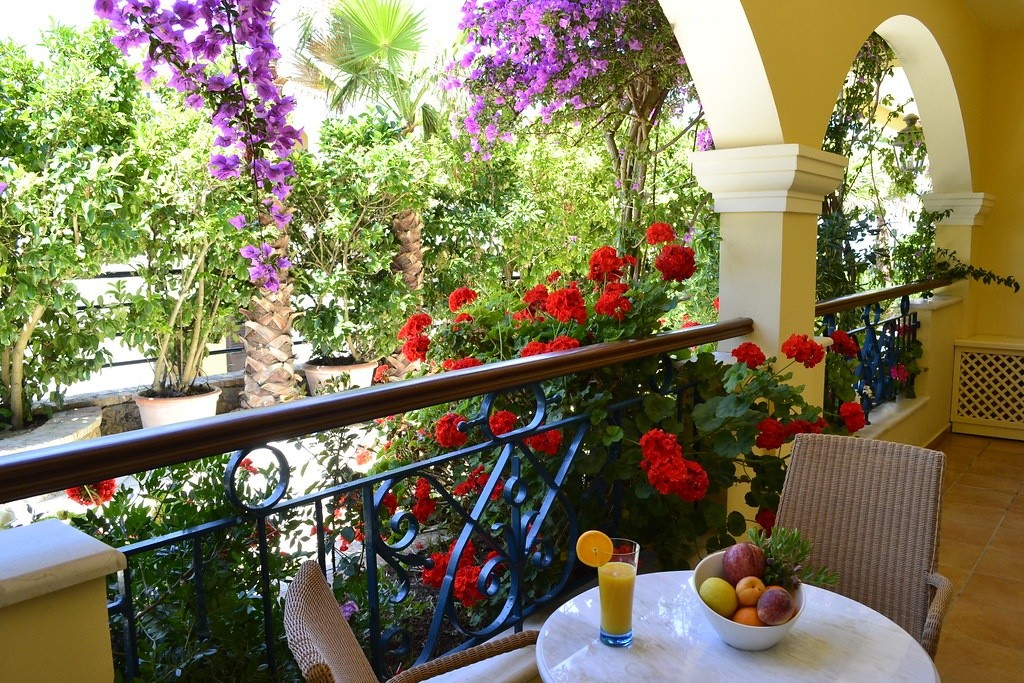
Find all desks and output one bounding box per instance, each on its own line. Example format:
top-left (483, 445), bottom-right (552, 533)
top-left (536, 570), bottom-right (943, 683)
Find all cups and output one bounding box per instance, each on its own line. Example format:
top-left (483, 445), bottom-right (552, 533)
top-left (595, 538), bottom-right (640, 647)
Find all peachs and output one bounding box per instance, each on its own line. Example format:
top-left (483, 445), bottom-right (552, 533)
top-left (757, 588), bottom-right (794, 625)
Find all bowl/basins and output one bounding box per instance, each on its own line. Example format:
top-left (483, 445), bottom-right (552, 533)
top-left (693, 551), bottom-right (807, 650)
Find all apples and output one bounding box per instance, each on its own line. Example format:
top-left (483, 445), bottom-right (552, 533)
top-left (722, 542), bottom-right (765, 583)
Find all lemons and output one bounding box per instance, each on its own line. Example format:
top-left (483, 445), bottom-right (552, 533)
top-left (576, 530), bottom-right (613, 567)
top-left (700, 577), bottom-right (737, 617)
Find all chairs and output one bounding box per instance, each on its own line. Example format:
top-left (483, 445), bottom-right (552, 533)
top-left (770, 434), bottom-right (953, 663)
top-left (284, 561), bottom-right (540, 682)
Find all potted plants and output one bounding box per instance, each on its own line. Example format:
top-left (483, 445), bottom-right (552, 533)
top-left (0, 13), bottom-right (454, 459)
